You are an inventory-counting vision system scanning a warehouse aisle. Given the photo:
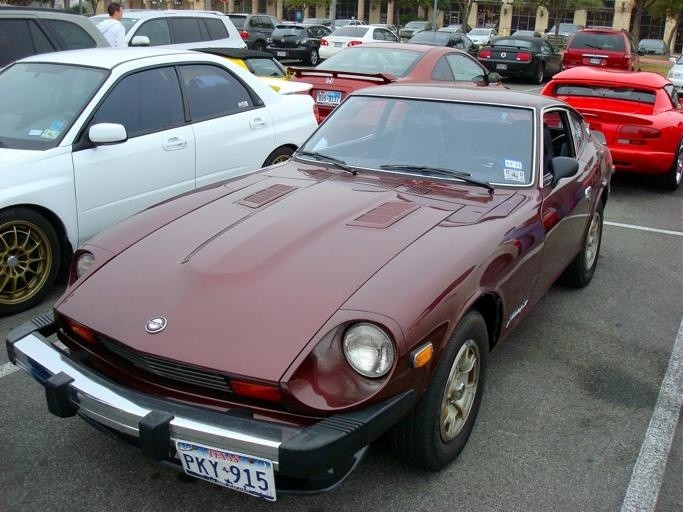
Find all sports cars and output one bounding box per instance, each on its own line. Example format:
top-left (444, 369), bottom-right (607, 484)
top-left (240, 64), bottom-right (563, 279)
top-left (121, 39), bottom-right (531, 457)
top-left (5, 83), bottom-right (612, 503)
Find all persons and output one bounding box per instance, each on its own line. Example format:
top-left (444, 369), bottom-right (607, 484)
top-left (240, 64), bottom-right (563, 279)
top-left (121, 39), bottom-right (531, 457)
top-left (95, 2), bottom-right (126, 48)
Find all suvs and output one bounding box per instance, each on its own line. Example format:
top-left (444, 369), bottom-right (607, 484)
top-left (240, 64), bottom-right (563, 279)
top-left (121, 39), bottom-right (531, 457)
top-left (543, 22), bottom-right (583, 49)
top-left (561, 26), bottom-right (640, 75)
top-left (85, 7), bottom-right (247, 52)
top-left (1, 4), bottom-right (114, 73)
top-left (226, 11), bottom-right (281, 58)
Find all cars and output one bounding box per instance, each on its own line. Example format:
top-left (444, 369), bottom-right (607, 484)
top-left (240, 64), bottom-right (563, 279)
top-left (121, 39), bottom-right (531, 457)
top-left (446, 22), bottom-right (472, 33)
top-left (537, 67), bottom-right (682, 192)
top-left (466, 27), bottom-right (500, 48)
top-left (284, 43), bottom-right (510, 144)
top-left (476, 34), bottom-right (562, 84)
top-left (270, 17), bottom-right (400, 65)
top-left (637, 38), bottom-right (668, 58)
top-left (511, 30), bottom-right (541, 37)
top-left (0, 46), bottom-right (323, 321)
top-left (398, 21), bottom-right (434, 39)
top-left (665, 52), bottom-right (682, 99)
top-left (407, 30), bottom-right (478, 58)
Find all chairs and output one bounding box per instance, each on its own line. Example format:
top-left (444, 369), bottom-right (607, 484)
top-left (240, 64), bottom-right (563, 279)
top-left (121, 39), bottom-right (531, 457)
top-left (503, 119), bottom-right (554, 175)
top-left (389, 110), bottom-right (446, 168)
